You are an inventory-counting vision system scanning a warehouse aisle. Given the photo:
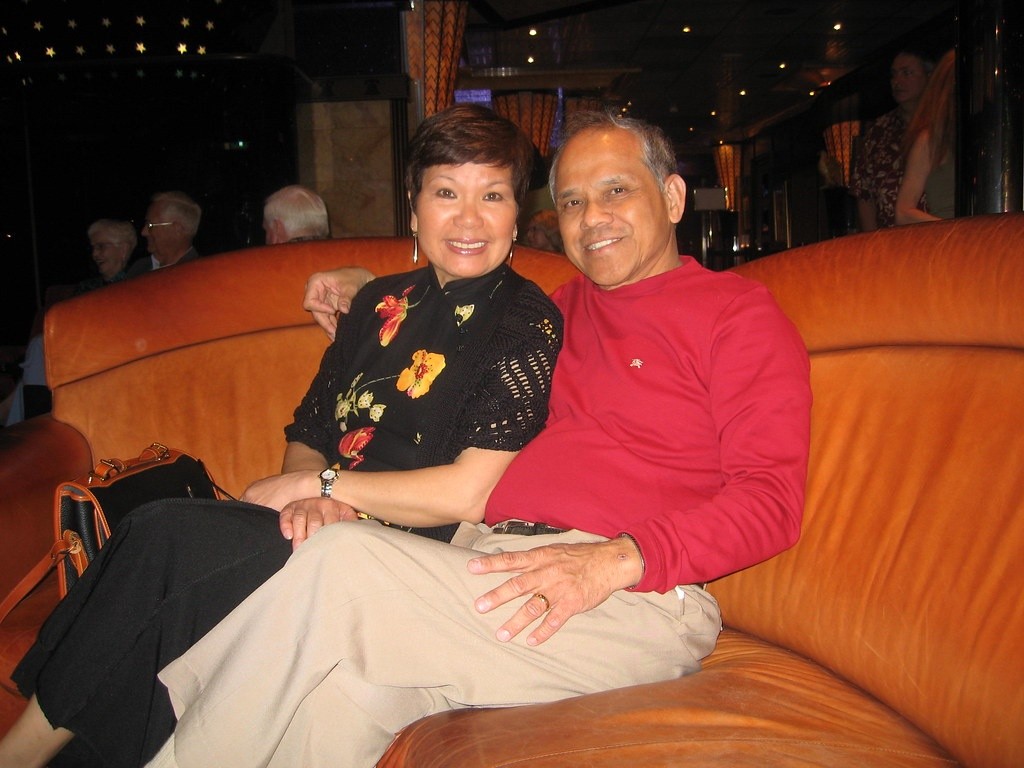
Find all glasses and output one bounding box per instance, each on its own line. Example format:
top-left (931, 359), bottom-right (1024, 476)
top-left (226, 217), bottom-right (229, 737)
top-left (144, 221), bottom-right (173, 229)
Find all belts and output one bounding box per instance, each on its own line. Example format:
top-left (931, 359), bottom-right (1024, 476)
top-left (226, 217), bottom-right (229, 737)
top-left (493, 520), bottom-right (567, 537)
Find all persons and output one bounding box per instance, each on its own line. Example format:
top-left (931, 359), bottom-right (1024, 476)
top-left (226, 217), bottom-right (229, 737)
top-left (852, 47), bottom-right (958, 229)
top-left (519, 183), bottom-right (564, 256)
top-left (262, 183), bottom-right (329, 244)
top-left (136, 115), bottom-right (813, 768)
top-left (0, 190), bottom-right (201, 429)
top-left (0, 105), bottom-right (565, 768)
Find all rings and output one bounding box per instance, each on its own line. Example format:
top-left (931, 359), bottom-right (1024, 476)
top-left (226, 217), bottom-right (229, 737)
top-left (533, 594), bottom-right (550, 609)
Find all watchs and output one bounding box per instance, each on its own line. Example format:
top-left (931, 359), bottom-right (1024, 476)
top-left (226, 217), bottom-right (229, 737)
top-left (317, 468), bottom-right (340, 498)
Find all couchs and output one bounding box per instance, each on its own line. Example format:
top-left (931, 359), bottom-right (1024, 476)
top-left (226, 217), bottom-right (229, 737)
top-left (40, 224), bottom-right (1023, 768)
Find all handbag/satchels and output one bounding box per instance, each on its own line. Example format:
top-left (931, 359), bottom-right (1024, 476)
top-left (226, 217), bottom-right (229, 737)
top-left (0, 441), bottom-right (238, 622)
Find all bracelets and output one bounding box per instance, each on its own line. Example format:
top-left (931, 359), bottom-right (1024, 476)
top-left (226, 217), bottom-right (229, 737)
top-left (618, 532), bottom-right (645, 590)
